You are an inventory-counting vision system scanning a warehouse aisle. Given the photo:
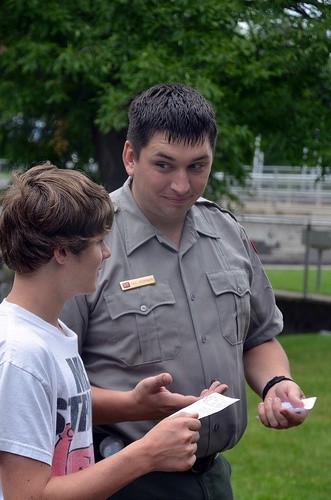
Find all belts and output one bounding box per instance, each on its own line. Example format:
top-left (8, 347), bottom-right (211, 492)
top-left (182, 452), bottom-right (220, 474)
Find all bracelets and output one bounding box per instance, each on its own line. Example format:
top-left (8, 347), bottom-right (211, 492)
top-left (262, 375), bottom-right (295, 402)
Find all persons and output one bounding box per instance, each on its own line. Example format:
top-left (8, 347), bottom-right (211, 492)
top-left (0, 164), bottom-right (201, 500)
top-left (58, 83), bottom-right (310, 500)
